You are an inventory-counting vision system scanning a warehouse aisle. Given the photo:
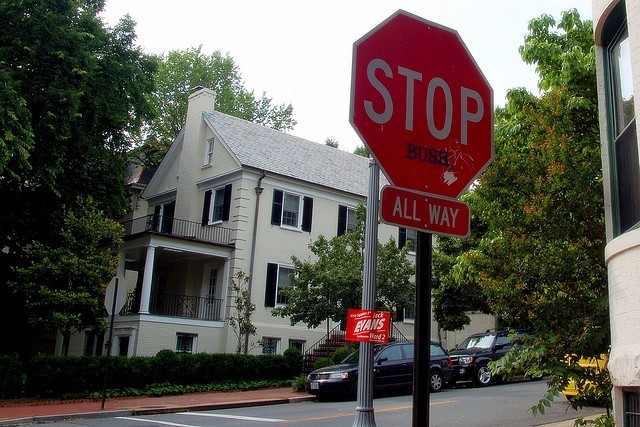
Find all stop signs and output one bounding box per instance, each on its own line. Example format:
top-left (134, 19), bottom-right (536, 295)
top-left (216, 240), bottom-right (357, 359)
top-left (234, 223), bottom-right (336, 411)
top-left (349, 9), bottom-right (495, 200)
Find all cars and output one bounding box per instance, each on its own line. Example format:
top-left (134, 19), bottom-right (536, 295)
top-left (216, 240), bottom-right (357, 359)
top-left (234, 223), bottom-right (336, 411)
top-left (305, 341), bottom-right (454, 401)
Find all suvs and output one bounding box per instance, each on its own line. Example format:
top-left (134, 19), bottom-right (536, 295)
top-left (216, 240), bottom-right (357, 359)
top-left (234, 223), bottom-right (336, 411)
top-left (448, 329), bottom-right (543, 385)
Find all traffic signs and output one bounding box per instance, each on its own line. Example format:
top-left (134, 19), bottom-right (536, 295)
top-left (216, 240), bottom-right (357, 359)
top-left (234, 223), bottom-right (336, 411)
top-left (378, 184), bottom-right (472, 238)
